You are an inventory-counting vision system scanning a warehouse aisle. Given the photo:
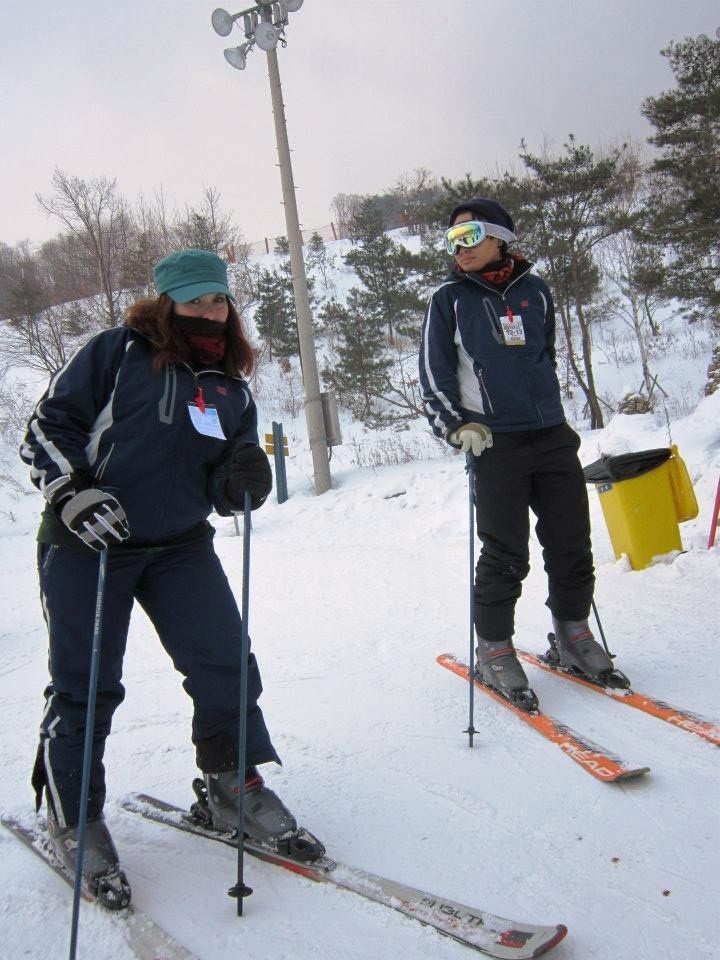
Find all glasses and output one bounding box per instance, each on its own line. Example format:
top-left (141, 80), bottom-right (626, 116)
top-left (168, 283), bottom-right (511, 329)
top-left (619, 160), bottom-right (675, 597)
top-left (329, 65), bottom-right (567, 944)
top-left (443, 221), bottom-right (485, 255)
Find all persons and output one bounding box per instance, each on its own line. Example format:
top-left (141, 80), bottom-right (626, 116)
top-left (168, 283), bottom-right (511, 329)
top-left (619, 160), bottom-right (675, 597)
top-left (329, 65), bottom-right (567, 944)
top-left (19, 251), bottom-right (296, 883)
top-left (418, 199), bottom-right (613, 690)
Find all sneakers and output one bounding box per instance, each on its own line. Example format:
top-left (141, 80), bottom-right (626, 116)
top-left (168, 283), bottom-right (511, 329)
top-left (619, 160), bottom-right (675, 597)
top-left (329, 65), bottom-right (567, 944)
top-left (43, 793), bottom-right (118, 877)
top-left (552, 617), bottom-right (611, 673)
top-left (204, 769), bottom-right (298, 840)
top-left (479, 635), bottom-right (527, 690)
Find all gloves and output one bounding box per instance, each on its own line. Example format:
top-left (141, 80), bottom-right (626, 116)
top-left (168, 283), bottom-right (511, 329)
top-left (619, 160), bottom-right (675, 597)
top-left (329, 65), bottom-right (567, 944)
top-left (229, 445), bottom-right (271, 496)
top-left (42, 475), bottom-right (131, 551)
top-left (451, 423), bottom-right (494, 456)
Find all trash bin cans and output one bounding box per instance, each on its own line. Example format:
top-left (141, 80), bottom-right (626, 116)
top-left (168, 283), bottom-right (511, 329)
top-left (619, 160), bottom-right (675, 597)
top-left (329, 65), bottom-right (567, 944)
top-left (582, 445), bottom-right (699, 570)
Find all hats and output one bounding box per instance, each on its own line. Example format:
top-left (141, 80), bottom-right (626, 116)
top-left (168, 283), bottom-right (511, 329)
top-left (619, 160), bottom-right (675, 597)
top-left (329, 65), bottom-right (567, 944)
top-left (155, 249), bottom-right (234, 304)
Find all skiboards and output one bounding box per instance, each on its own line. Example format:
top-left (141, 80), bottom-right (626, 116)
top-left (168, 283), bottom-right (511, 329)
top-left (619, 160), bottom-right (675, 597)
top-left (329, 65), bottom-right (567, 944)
top-left (434, 643), bottom-right (719, 786)
top-left (0, 791), bottom-right (570, 960)
